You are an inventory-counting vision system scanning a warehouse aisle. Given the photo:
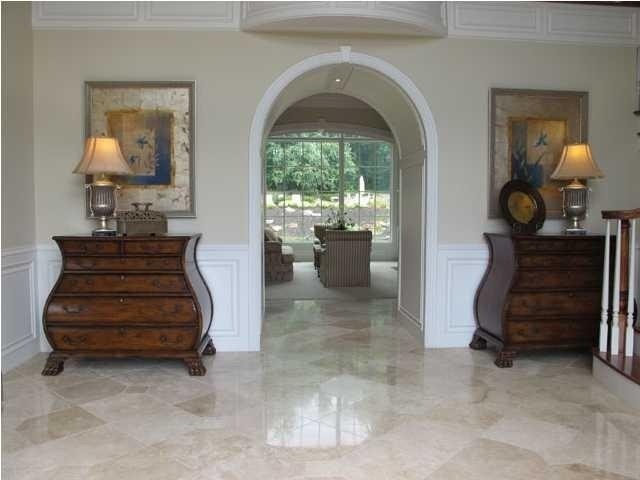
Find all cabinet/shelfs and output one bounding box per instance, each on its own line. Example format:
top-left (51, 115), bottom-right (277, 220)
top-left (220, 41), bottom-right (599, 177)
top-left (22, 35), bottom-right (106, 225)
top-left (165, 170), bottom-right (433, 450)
top-left (468, 232), bottom-right (638, 368)
top-left (40, 232), bottom-right (217, 376)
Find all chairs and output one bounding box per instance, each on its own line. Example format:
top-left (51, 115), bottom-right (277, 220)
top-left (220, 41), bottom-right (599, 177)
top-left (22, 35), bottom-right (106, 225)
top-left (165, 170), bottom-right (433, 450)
top-left (264, 227), bottom-right (294, 282)
top-left (313, 223), bottom-right (373, 288)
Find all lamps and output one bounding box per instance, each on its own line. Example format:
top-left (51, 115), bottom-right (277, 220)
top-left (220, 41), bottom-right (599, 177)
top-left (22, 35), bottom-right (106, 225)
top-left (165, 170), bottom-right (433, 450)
top-left (72, 135), bottom-right (134, 237)
top-left (549, 143), bottom-right (605, 235)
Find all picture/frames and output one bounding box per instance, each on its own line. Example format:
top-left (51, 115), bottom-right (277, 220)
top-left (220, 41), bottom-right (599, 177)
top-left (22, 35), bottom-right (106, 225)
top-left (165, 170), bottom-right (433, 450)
top-left (83, 80), bottom-right (197, 219)
top-left (486, 87), bottom-right (590, 219)
